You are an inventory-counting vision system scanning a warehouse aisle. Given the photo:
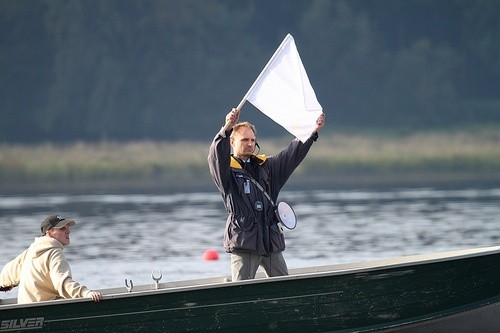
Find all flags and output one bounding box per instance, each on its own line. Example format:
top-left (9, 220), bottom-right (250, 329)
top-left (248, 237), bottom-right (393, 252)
top-left (245, 34), bottom-right (324, 144)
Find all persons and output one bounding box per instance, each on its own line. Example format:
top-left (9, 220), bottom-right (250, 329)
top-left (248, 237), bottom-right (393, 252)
top-left (208, 107), bottom-right (323, 282)
top-left (0, 214), bottom-right (102, 303)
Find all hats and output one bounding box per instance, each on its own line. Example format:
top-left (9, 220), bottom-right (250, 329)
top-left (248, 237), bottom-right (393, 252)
top-left (40, 215), bottom-right (76, 234)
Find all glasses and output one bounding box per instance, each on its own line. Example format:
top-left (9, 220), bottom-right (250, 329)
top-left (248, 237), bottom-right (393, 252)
top-left (57, 226), bottom-right (70, 232)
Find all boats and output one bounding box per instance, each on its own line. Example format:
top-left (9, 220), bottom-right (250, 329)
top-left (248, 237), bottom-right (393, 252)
top-left (2, 244), bottom-right (499, 333)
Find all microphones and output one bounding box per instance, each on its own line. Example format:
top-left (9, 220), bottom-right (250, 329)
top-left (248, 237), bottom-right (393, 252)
top-left (251, 142), bottom-right (260, 159)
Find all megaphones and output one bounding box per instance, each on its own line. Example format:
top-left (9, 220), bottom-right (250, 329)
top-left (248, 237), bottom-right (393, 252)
top-left (277, 200), bottom-right (297, 232)
